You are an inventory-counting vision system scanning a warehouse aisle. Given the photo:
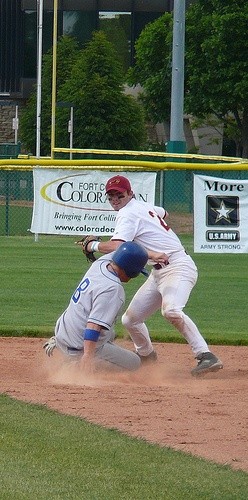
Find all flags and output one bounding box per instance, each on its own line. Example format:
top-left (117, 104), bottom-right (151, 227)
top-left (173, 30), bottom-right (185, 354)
top-left (30, 168), bottom-right (158, 237)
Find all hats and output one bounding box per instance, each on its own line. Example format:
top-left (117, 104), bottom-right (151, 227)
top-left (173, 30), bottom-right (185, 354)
top-left (103, 175), bottom-right (131, 193)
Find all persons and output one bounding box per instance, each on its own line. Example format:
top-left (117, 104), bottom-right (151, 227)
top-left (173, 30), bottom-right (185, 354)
top-left (54, 241), bottom-right (170, 383)
top-left (75, 175), bottom-right (224, 376)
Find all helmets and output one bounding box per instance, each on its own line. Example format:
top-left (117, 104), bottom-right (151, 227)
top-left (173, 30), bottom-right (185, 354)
top-left (111, 241), bottom-right (149, 278)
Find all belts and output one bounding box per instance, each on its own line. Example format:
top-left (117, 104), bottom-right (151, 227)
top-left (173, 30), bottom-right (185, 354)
top-left (153, 251), bottom-right (188, 269)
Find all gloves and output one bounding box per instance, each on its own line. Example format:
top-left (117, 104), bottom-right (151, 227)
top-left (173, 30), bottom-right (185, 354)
top-left (43, 336), bottom-right (57, 358)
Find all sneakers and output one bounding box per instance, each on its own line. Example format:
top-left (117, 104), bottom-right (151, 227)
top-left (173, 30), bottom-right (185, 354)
top-left (135, 352), bottom-right (157, 366)
top-left (190, 352), bottom-right (224, 376)
